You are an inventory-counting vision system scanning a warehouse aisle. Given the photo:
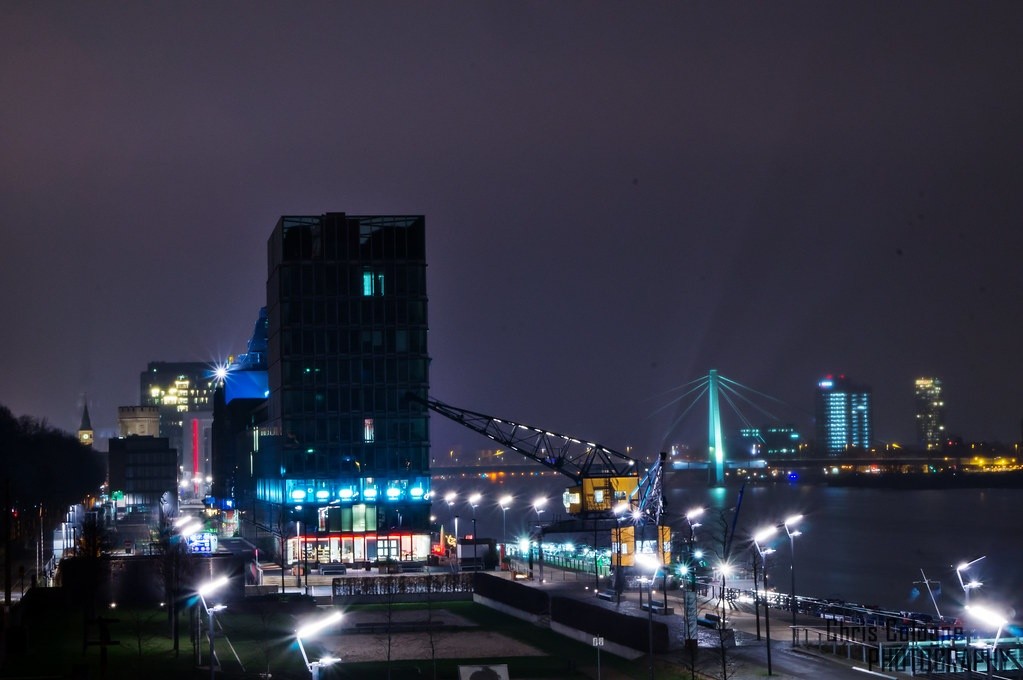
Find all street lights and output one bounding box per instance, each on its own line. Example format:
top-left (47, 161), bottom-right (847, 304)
top-left (753, 536), bottom-right (775, 676)
top-left (533, 496), bottom-right (545, 580)
top-left (469, 493), bottom-right (479, 572)
top-left (61, 505), bottom-right (79, 558)
top-left (199, 577), bottom-right (228, 680)
top-left (614, 503), bottom-right (641, 604)
top-left (784, 518), bottom-right (802, 646)
top-left (686, 507), bottom-right (703, 591)
top-left (499, 495), bottom-right (509, 545)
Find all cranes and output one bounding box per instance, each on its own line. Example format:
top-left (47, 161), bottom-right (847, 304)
top-left (404, 392), bottom-right (642, 518)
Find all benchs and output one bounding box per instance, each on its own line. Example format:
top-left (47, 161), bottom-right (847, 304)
top-left (400, 568), bottom-right (422, 573)
top-left (642, 600), bottom-right (674, 615)
top-left (697, 613), bottom-right (731, 630)
top-left (597, 588), bottom-right (627, 602)
top-left (322, 569), bottom-right (345, 575)
